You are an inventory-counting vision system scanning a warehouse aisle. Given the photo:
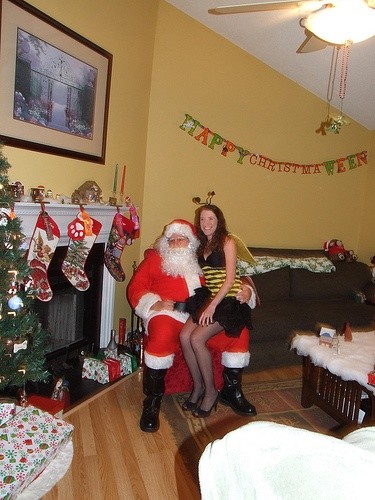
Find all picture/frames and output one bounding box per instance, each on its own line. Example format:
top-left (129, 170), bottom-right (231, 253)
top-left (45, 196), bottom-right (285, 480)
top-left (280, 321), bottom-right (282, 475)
top-left (0, 0), bottom-right (114, 165)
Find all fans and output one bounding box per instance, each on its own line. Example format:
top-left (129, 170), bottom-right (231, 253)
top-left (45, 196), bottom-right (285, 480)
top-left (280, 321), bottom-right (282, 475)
top-left (207, 0), bottom-right (335, 53)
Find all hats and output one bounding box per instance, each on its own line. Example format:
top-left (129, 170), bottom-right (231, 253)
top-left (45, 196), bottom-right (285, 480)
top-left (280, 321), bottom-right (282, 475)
top-left (164, 219), bottom-right (196, 240)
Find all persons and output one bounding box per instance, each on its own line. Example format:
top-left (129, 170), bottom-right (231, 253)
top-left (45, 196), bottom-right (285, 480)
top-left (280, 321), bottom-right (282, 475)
top-left (179, 191), bottom-right (257, 419)
top-left (127, 219), bottom-right (256, 431)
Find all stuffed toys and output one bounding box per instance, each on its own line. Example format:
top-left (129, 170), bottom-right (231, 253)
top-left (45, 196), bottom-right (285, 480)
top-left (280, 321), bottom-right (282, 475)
top-left (78, 181), bottom-right (102, 202)
top-left (324, 239), bottom-right (357, 262)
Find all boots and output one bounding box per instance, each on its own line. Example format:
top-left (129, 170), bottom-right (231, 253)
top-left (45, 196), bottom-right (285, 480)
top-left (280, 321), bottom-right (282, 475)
top-left (218, 367), bottom-right (256, 416)
top-left (139, 364), bottom-right (168, 433)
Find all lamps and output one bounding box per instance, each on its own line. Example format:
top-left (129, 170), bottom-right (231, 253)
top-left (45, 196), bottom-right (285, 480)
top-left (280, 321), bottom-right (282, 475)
top-left (298, 0), bottom-right (375, 134)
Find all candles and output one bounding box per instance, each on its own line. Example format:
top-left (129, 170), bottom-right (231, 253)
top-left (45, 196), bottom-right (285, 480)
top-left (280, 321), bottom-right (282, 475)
top-left (121, 164), bottom-right (126, 193)
top-left (113, 163), bottom-right (119, 193)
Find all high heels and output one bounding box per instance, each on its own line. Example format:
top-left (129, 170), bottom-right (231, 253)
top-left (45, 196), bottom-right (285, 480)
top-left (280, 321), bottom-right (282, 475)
top-left (192, 391), bottom-right (220, 418)
top-left (182, 389), bottom-right (205, 411)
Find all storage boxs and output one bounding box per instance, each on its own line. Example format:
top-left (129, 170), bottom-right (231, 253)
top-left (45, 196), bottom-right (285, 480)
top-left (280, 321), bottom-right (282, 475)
top-left (0, 400), bottom-right (75, 500)
top-left (82, 353), bottom-right (137, 384)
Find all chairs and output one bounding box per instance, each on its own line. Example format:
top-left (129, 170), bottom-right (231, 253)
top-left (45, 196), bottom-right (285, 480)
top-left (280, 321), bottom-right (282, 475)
top-left (144, 249), bottom-right (224, 395)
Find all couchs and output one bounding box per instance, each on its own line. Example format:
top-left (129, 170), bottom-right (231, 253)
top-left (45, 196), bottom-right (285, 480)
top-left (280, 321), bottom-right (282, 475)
top-left (236, 247), bottom-right (375, 375)
top-left (197, 421), bottom-right (375, 500)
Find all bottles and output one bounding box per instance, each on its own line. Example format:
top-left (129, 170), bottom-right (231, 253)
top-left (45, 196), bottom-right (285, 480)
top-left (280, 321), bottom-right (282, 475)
top-left (106, 329), bottom-right (118, 351)
top-left (344, 322), bottom-right (352, 342)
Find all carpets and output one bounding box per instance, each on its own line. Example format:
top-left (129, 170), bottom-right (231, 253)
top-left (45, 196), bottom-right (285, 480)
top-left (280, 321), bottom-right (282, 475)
top-left (14, 436), bottom-right (74, 500)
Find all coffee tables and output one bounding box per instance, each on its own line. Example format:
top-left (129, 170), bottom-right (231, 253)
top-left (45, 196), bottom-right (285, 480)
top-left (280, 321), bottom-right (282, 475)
top-left (291, 331), bottom-right (375, 432)
